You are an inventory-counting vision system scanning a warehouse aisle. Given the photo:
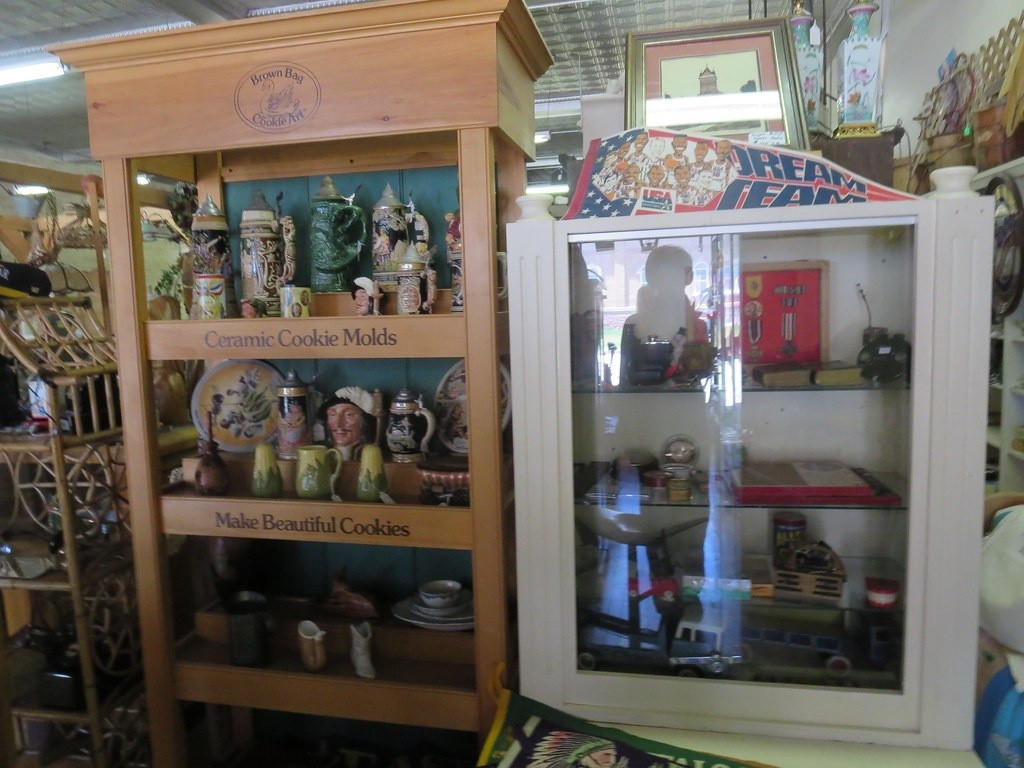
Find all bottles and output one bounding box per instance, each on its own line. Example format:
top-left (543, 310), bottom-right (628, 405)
top-left (239, 187), bottom-right (296, 297)
top-left (191, 194), bottom-right (233, 281)
top-left (772, 511), bottom-right (807, 569)
top-left (372, 181), bottom-right (429, 283)
top-left (309, 175), bottom-right (369, 292)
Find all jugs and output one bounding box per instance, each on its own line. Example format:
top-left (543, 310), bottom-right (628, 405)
top-left (385, 384), bottom-right (436, 464)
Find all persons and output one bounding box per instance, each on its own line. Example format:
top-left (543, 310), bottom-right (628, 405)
top-left (316, 384), bottom-right (381, 463)
top-left (618, 245), bottom-right (713, 644)
top-left (349, 277), bottom-right (388, 318)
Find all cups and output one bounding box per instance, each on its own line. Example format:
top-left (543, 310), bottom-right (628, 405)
top-left (297, 620), bottom-right (326, 671)
top-left (395, 262), bottom-right (437, 315)
top-left (660, 463), bottom-right (696, 501)
top-left (193, 273), bottom-right (227, 321)
top-left (419, 580), bottom-right (462, 609)
top-left (227, 591), bottom-right (273, 669)
top-left (295, 444), bottom-right (343, 501)
top-left (278, 286), bottom-right (310, 317)
top-left (450, 251), bottom-right (508, 312)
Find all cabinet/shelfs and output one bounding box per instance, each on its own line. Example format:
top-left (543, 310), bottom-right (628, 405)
top-left (48, 1), bottom-right (553, 768)
top-left (972, 158), bottom-right (1024, 494)
top-left (504, 168), bottom-right (995, 755)
top-left (0, 297), bottom-right (151, 768)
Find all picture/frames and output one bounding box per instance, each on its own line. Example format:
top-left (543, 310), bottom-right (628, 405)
top-left (622, 16), bottom-right (810, 159)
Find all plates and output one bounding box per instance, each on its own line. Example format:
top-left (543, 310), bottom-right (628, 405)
top-left (391, 600), bottom-right (474, 631)
top-left (406, 597), bottom-right (474, 623)
